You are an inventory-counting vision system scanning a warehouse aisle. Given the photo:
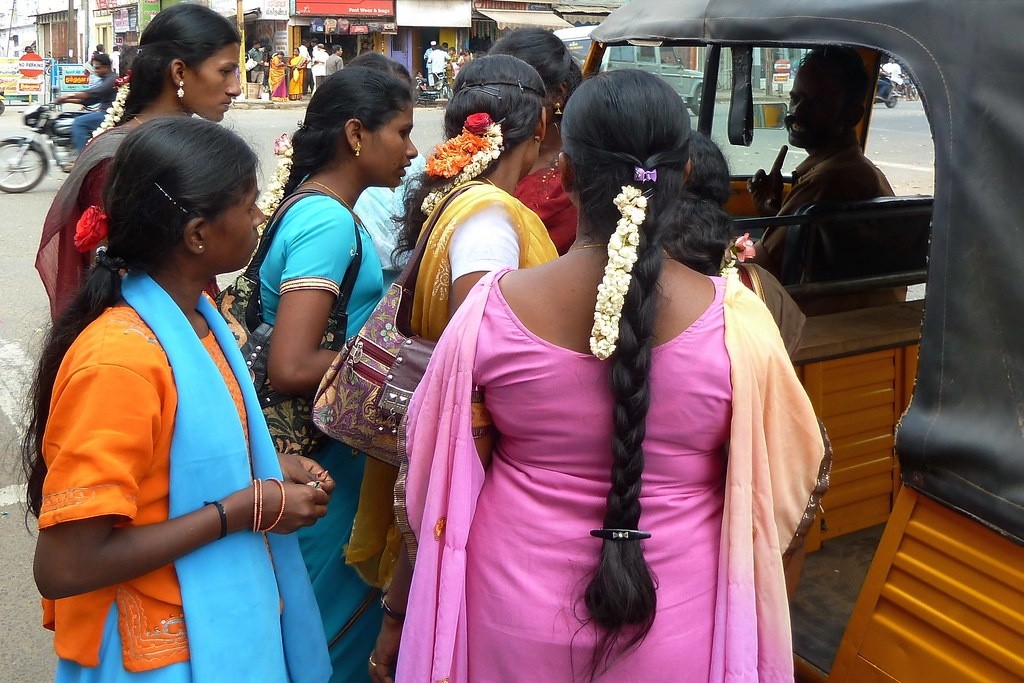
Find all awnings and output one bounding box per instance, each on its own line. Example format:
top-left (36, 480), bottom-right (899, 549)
top-left (553, 4), bottom-right (619, 25)
top-left (476, 8), bottom-right (575, 32)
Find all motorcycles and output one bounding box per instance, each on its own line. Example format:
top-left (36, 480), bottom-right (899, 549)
top-left (522, 1), bottom-right (1024, 683)
top-left (0, 87), bottom-right (94, 193)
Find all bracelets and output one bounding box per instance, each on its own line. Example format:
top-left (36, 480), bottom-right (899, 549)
top-left (204, 500), bottom-right (227, 540)
top-left (253, 478), bottom-right (263, 533)
top-left (259, 477), bottom-right (286, 532)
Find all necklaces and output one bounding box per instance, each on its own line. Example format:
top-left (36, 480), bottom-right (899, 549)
top-left (300, 181), bottom-right (348, 207)
top-left (133, 115), bottom-right (143, 124)
top-left (483, 176), bottom-right (495, 186)
top-left (573, 243), bottom-right (667, 251)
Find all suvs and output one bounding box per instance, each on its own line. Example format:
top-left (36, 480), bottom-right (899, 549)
top-left (554, 25), bottom-right (705, 118)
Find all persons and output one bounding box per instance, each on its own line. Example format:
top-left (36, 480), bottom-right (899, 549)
top-left (216, 67), bottom-right (419, 683)
top-left (486, 27), bottom-right (582, 257)
top-left (369, 67), bottom-right (833, 683)
top-left (346, 52), bottom-right (426, 296)
top-left (312, 54), bottom-right (561, 593)
top-left (740, 45), bottom-right (908, 317)
top-left (20, 116), bottom-right (334, 683)
top-left (26, 42), bottom-right (487, 173)
top-left (34, 4), bottom-right (241, 327)
top-left (662, 130), bottom-right (806, 362)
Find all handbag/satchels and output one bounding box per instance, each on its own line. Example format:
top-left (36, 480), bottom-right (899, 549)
top-left (215, 190), bottom-right (362, 455)
top-left (312, 182), bottom-right (482, 468)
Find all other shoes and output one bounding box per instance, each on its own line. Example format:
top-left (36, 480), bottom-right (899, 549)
top-left (64, 162), bottom-right (74, 172)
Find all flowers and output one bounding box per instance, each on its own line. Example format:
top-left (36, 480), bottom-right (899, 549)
top-left (719, 233), bottom-right (756, 274)
top-left (73, 205), bottom-right (110, 253)
top-left (248, 133), bottom-right (292, 259)
top-left (85, 83), bottom-right (134, 147)
top-left (589, 185), bottom-right (650, 360)
top-left (420, 114), bottom-right (505, 216)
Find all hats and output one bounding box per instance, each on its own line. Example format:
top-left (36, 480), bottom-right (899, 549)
top-left (431, 41), bottom-right (436, 46)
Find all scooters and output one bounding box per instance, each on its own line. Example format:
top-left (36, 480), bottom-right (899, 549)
top-left (412, 67), bottom-right (455, 107)
top-left (875, 64), bottom-right (920, 108)
